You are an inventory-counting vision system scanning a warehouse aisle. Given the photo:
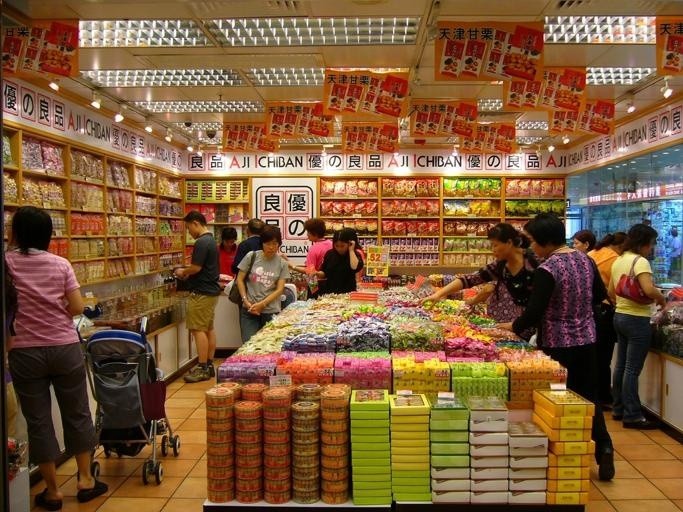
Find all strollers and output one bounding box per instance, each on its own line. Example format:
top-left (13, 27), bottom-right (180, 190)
top-left (76, 315), bottom-right (180, 485)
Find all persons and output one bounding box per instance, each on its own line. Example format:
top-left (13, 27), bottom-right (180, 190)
top-left (4, 207), bottom-right (107, 512)
top-left (570, 227), bottom-right (595, 255)
top-left (307, 229), bottom-right (361, 296)
top-left (466, 277), bottom-right (526, 323)
top-left (173, 212), bottom-right (220, 383)
top-left (607, 224), bottom-right (666, 430)
top-left (235, 224), bottom-right (290, 345)
top-left (585, 231), bottom-right (628, 412)
top-left (349, 227), bottom-right (367, 267)
top-left (232, 218), bottom-right (265, 276)
top-left (519, 220), bottom-right (545, 266)
top-left (514, 211), bottom-right (615, 479)
top-left (417, 223), bottom-right (538, 337)
top-left (215, 226), bottom-right (238, 290)
top-left (4, 255), bottom-right (18, 438)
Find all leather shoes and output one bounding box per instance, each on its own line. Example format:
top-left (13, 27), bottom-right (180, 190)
top-left (597, 444), bottom-right (615, 480)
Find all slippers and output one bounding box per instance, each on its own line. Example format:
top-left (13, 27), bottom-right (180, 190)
top-left (35, 487), bottom-right (63, 511)
top-left (75, 477), bottom-right (109, 502)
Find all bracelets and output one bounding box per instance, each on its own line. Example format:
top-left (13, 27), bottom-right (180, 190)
top-left (240, 297), bottom-right (246, 301)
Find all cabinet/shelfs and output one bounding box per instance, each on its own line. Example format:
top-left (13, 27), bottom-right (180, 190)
top-left (184, 172), bottom-right (565, 350)
top-left (2, 117), bottom-right (184, 486)
top-left (611, 339), bottom-right (682, 434)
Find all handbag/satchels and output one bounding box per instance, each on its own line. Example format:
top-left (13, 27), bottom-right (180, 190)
top-left (227, 251), bottom-right (257, 306)
top-left (614, 254), bottom-right (655, 306)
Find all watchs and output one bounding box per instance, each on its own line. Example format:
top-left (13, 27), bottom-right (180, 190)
top-left (282, 219), bottom-right (332, 277)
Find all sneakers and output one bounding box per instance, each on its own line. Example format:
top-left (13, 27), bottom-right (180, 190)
top-left (611, 408), bottom-right (623, 421)
top-left (189, 363), bottom-right (216, 378)
top-left (183, 368), bottom-right (211, 382)
top-left (622, 413), bottom-right (660, 430)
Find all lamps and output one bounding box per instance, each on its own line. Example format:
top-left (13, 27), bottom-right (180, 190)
top-left (48, 71), bottom-right (207, 156)
top-left (535, 71), bottom-right (674, 158)
top-left (216, 145), bottom-right (334, 157)
